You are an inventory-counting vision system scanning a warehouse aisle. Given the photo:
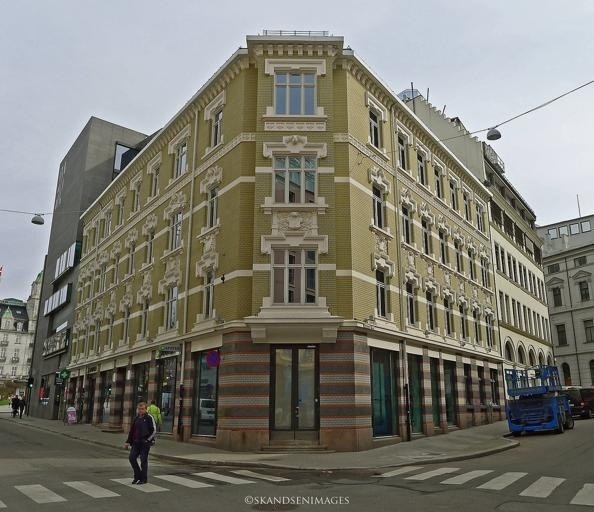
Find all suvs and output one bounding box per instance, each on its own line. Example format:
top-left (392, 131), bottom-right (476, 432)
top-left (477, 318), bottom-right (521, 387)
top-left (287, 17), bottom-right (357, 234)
top-left (561, 386), bottom-right (594, 418)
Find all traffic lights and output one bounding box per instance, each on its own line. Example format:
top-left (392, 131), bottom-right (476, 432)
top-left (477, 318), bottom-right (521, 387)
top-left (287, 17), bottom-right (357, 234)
top-left (27, 378), bottom-right (35, 389)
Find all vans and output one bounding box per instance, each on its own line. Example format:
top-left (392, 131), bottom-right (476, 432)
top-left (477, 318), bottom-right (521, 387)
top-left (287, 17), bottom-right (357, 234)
top-left (199, 399), bottom-right (216, 425)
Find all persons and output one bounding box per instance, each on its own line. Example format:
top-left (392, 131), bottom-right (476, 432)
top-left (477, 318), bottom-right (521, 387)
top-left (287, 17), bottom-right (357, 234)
top-left (147, 400), bottom-right (164, 446)
top-left (8, 394), bottom-right (25, 420)
top-left (123, 402), bottom-right (157, 485)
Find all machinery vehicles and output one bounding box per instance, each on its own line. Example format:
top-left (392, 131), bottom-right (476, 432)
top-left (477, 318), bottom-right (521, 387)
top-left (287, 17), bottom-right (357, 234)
top-left (505, 365), bottom-right (574, 434)
